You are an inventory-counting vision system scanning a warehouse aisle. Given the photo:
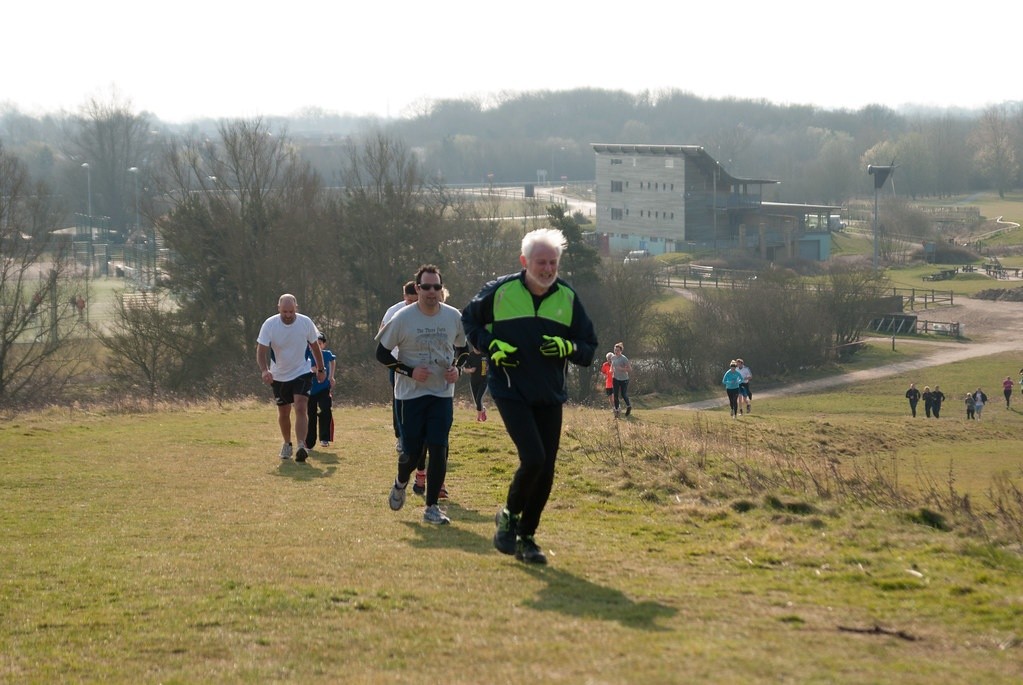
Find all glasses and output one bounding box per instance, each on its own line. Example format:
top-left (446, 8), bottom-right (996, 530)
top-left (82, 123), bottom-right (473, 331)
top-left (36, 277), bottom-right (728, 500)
top-left (417, 283), bottom-right (442, 290)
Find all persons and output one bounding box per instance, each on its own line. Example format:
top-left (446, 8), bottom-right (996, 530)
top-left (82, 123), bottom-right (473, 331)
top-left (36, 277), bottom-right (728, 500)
top-left (70, 294), bottom-right (85, 317)
top-left (256, 294), bottom-right (337, 464)
top-left (932, 385), bottom-right (945, 418)
top-left (461, 228), bottom-right (598, 564)
top-left (375, 264), bottom-right (469, 528)
top-left (965, 393), bottom-right (975, 420)
top-left (906, 384), bottom-right (920, 418)
top-left (922, 386), bottom-right (936, 418)
top-left (972, 387), bottom-right (987, 421)
top-left (601, 343), bottom-right (632, 418)
top-left (722, 359), bottom-right (753, 420)
top-left (467, 347), bottom-right (488, 422)
top-left (1002, 376), bottom-right (1014, 409)
top-left (1018, 368), bottom-right (1023, 395)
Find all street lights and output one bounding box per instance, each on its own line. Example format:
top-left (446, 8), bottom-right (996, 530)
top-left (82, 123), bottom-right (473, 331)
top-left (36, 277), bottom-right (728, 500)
top-left (81, 163), bottom-right (92, 240)
top-left (128, 166), bottom-right (140, 277)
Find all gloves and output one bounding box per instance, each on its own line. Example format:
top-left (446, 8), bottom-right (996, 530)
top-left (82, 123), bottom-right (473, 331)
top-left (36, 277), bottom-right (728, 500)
top-left (488, 338), bottom-right (520, 387)
top-left (539, 334), bottom-right (577, 360)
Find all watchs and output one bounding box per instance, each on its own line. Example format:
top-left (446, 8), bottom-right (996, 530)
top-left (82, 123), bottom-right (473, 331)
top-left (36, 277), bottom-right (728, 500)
top-left (318, 367), bottom-right (326, 373)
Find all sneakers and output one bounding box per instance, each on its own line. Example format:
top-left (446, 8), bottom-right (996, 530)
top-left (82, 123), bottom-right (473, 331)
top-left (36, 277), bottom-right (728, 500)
top-left (279, 442), bottom-right (293, 459)
top-left (413, 472), bottom-right (426, 494)
top-left (494, 509), bottom-right (521, 554)
top-left (423, 504), bottom-right (451, 524)
top-left (438, 484), bottom-right (449, 500)
top-left (294, 440), bottom-right (308, 463)
top-left (515, 540), bottom-right (547, 565)
top-left (388, 476), bottom-right (409, 511)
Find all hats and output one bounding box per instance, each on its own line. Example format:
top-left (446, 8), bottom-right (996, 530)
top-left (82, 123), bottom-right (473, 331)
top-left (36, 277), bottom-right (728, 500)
top-left (318, 332), bottom-right (326, 344)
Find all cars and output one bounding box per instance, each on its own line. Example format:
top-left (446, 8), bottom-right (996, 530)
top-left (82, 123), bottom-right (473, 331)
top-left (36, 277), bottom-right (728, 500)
top-left (623, 250), bottom-right (647, 266)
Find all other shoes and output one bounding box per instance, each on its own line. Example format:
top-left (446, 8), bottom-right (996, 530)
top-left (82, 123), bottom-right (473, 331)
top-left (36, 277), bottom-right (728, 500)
top-left (730, 409), bottom-right (733, 416)
top-left (746, 404), bottom-right (750, 413)
top-left (739, 413), bottom-right (742, 416)
top-left (733, 415), bottom-right (736, 419)
top-left (320, 440), bottom-right (329, 447)
top-left (625, 405), bottom-right (631, 416)
top-left (305, 448), bottom-right (312, 452)
top-left (614, 409), bottom-right (621, 417)
top-left (396, 437), bottom-right (403, 454)
top-left (477, 406), bottom-right (487, 421)
